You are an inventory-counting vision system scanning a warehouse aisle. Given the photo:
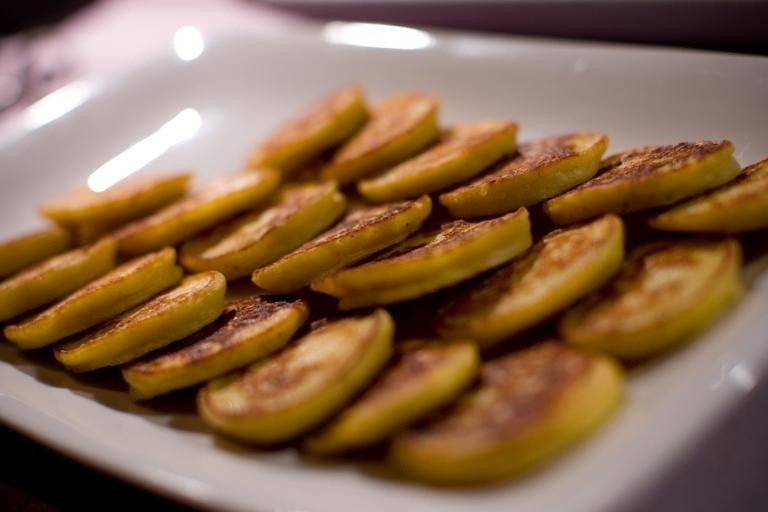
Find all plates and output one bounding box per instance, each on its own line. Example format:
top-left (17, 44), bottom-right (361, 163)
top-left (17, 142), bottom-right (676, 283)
top-left (0, 2), bottom-right (768, 512)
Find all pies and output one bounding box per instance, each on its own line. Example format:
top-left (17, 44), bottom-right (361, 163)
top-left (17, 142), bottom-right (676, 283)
top-left (0, 83), bottom-right (768, 484)
